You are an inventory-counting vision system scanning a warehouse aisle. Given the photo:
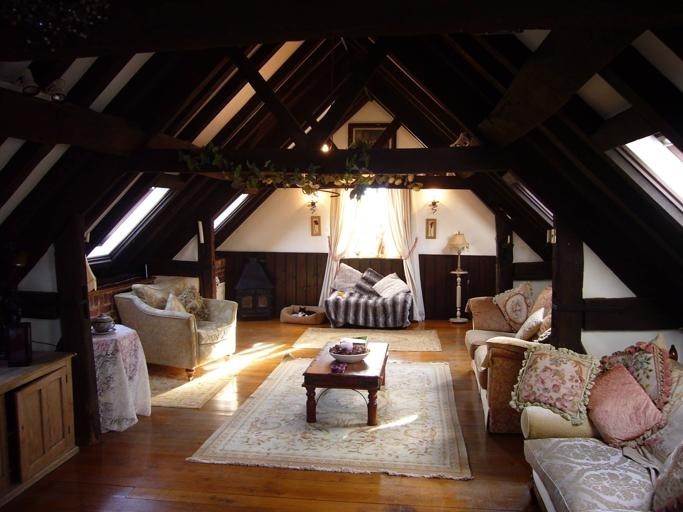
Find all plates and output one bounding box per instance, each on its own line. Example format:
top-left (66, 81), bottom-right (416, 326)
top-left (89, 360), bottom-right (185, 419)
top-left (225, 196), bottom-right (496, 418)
top-left (90, 328), bottom-right (117, 335)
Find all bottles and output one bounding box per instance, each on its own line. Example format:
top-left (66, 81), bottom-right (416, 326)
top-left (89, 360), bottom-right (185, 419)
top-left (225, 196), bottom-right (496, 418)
top-left (668, 344), bottom-right (678, 362)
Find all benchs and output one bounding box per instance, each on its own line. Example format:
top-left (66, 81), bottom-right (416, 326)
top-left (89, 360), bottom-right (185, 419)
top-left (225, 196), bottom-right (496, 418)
top-left (325, 291), bottom-right (412, 328)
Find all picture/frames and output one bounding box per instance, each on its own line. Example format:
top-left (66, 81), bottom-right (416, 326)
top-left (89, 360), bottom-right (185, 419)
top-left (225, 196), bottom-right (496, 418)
top-left (311, 216), bottom-right (321, 236)
top-left (426, 219), bottom-right (436, 239)
top-left (348, 122), bottom-right (396, 150)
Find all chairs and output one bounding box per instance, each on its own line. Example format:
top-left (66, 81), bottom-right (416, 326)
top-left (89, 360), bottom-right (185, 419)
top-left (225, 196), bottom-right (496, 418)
top-left (114, 292), bottom-right (238, 383)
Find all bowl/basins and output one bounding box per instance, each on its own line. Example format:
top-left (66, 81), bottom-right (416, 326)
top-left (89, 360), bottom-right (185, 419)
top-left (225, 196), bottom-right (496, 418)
top-left (330, 349), bottom-right (371, 364)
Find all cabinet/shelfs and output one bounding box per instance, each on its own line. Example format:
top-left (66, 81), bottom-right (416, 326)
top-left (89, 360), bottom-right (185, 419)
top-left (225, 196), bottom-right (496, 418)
top-left (3, 366), bottom-right (66, 487)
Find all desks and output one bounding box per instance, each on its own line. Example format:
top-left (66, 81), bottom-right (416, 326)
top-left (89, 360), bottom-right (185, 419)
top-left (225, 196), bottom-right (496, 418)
top-left (92, 323), bottom-right (151, 434)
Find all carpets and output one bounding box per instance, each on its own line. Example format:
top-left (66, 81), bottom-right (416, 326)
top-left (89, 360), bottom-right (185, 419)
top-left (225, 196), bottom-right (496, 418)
top-left (292, 327), bottom-right (442, 352)
top-left (185, 353), bottom-right (474, 482)
top-left (148, 355), bottom-right (243, 410)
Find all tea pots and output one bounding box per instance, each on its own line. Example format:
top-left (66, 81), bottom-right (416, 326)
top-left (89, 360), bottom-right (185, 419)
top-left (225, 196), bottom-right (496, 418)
top-left (90, 313), bottom-right (116, 332)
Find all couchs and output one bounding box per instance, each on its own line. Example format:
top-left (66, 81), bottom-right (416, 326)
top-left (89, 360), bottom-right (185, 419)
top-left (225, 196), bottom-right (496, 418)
top-left (520, 334), bottom-right (683, 511)
top-left (464, 297), bottom-right (552, 435)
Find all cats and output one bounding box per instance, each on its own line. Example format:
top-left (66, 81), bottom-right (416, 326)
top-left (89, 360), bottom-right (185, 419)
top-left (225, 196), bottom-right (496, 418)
top-left (298, 306), bottom-right (316, 318)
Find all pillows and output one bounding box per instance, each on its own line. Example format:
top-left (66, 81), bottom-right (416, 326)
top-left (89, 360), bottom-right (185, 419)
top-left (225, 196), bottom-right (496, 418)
top-left (588, 364), bottom-right (666, 447)
top-left (132, 278), bottom-right (185, 310)
top-left (600, 341), bottom-right (670, 411)
top-left (164, 294), bottom-right (186, 312)
top-left (331, 264), bottom-right (362, 293)
top-left (354, 268), bottom-right (382, 296)
top-left (373, 273), bottom-right (410, 298)
top-left (493, 283), bottom-right (552, 339)
top-left (177, 285), bottom-right (208, 322)
top-left (511, 342), bottom-right (598, 426)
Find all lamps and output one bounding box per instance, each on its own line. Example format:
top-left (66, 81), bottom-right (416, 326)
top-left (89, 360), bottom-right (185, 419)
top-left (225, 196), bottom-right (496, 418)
top-left (446, 231), bottom-right (469, 323)
top-left (429, 201), bottom-right (439, 214)
top-left (308, 200), bottom-right (317, 213)
